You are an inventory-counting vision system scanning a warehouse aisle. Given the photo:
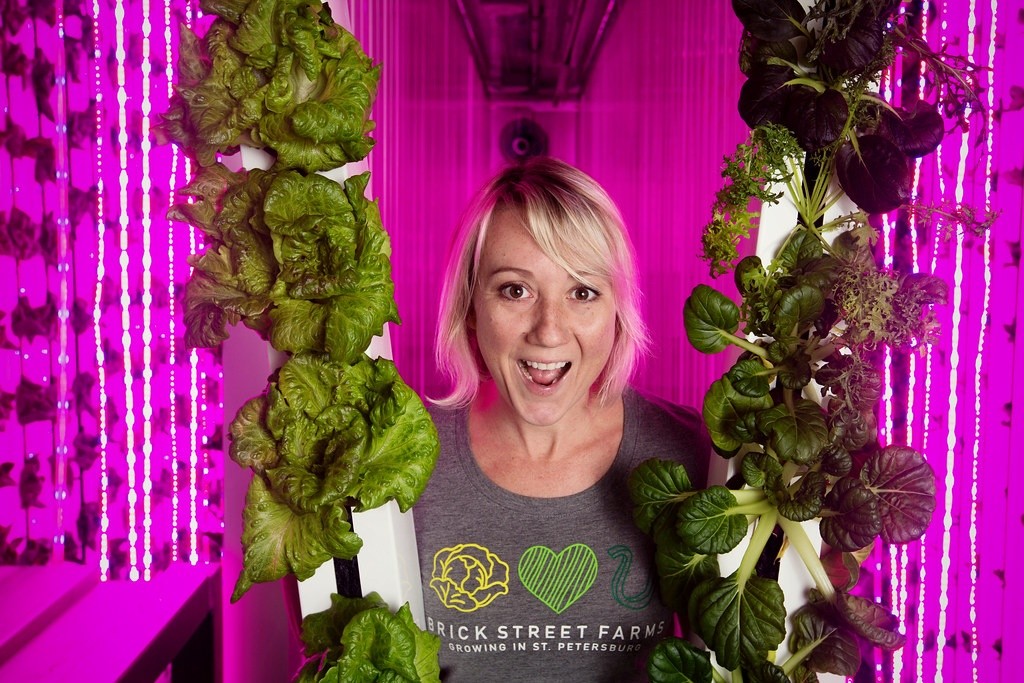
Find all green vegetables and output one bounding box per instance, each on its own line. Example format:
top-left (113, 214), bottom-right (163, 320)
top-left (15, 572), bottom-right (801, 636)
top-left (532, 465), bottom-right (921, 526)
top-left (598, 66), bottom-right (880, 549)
top-left (160, 0), bottom-right (1000, 682)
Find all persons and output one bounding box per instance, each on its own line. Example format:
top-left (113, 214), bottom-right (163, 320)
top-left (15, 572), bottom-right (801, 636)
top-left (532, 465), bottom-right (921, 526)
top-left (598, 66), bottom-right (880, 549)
top-left (412, 158), bottom-right (711, 683)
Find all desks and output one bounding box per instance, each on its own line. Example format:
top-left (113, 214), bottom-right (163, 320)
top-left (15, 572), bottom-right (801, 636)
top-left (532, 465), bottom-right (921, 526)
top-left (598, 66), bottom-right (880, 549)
top-left (0, 559), bottom-right (224, 683)
